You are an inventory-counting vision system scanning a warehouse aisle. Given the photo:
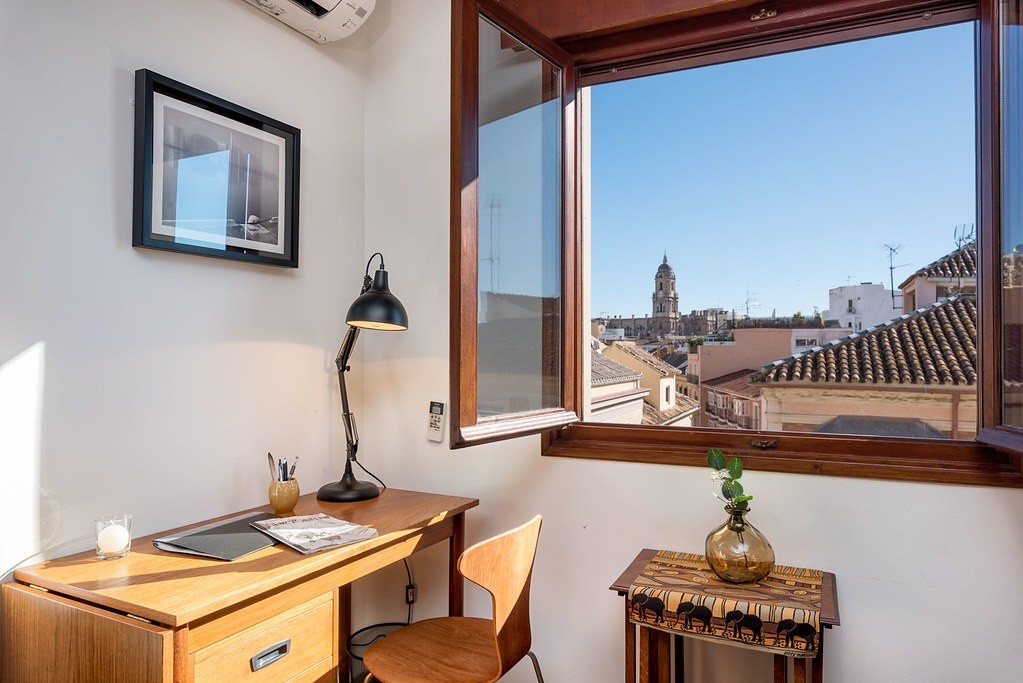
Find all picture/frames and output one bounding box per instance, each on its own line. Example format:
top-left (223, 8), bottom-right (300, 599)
top-left (131, 69), bottom-right (300, 269)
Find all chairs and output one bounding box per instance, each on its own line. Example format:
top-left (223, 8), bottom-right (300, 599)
top-left (364, 514), bottom-right (544, 683)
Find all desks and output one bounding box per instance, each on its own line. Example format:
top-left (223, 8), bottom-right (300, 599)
top-left (607, 549), bottom-right (840, 683)
top-left (1, 486), bottom-right (479, 683)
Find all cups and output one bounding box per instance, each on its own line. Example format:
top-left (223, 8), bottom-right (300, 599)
top-left (95, 514), bottom-right (134, 560)
top-left (268, 478), bottom-right (300, 513)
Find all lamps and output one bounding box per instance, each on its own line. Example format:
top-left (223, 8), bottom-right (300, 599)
top-left (317, 253), bottom-right (408, 503)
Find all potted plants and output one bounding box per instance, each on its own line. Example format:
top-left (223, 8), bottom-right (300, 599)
top-left (705, 448), bottom-right (775, 584)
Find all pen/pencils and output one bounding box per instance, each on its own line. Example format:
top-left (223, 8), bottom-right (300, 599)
top-left (264, 451), bottom-right (299, 482)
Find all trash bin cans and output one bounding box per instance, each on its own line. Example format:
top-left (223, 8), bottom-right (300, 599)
top-left (345, 621), bottom-right (411, 683)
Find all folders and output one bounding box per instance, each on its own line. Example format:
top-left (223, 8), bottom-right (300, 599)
top-left (151, 507), bottom-right (290, 561)
